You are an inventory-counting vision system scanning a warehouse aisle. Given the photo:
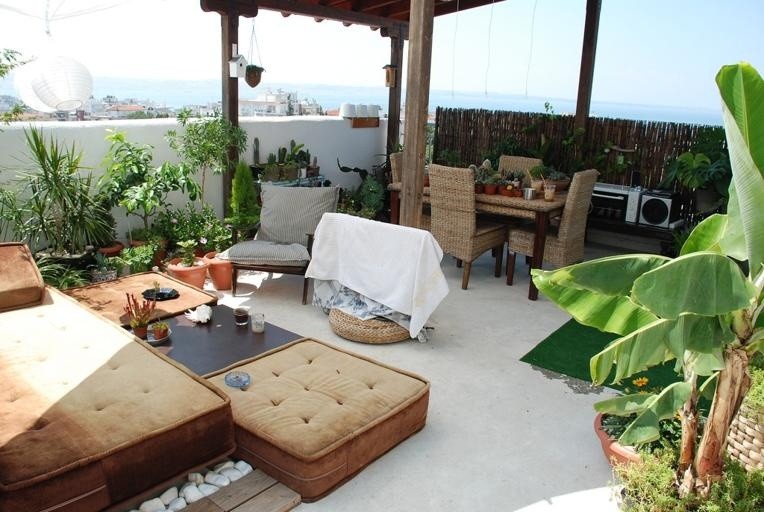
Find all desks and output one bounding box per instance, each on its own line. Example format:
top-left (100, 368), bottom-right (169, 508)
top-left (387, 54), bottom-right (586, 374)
top-left (388, 182), bottom-right (568, 300)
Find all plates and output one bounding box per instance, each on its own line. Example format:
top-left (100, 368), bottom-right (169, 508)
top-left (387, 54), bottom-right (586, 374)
top-left (224, 371), bottom-right (250, 388)
top-left (130, 324), bottom-right (172, 345)
top-left (141, 287), bottom-right (178, 300)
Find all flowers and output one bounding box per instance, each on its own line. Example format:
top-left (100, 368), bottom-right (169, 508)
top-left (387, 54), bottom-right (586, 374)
top-left (628, 376), bottom-right (649, 395)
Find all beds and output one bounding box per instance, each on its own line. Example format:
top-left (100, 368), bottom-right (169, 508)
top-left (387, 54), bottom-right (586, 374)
top-left (0, 241), bottom-right (236, 512)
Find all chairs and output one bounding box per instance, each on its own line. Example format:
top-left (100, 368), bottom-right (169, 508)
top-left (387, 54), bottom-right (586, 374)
top-left (231, 179), bottom-right (332, 304)
top-left (390, 151), bottom-right (600, 289)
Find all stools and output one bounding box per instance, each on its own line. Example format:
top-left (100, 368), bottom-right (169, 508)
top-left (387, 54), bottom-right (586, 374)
top-left (201, 336), bottom-right (430, 502)
top-left (61, 271), bottom-right (218, 331)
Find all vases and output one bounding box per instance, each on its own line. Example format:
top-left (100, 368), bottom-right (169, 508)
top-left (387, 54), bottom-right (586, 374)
top-left (594, 410), bottom-right (663, 482)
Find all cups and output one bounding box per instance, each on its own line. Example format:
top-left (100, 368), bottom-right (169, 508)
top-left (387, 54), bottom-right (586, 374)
top-left (531, 179), bottom-right (543, 194)
top-left (523, 188), bottom-right (537, 200)
top-left (250, 314), bottom-right (265, 332)
top-left (232, 307), bottom-right (249, 326)
top-left (543, 185), bottom-right (557, 202)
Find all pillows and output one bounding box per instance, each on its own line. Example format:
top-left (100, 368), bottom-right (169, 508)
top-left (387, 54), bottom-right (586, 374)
top-left (254, 182), bottom-right (341, 247)
top-left (216, 241), bottom-right (311, 267)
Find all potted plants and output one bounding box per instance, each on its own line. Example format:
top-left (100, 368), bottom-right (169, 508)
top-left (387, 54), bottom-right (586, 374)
top-left (122, 281), bottom-right (159, 338)
top-left (658, 127), bottom-right (732, 212)
top-left (153, 317), bottom-right (168, 339)
